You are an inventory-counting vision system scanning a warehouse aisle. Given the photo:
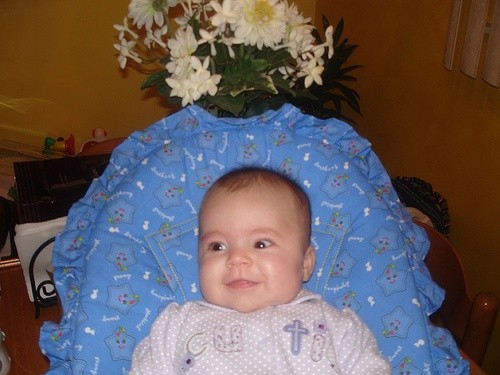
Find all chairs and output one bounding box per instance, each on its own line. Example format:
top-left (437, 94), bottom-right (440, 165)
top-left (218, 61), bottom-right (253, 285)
top-left (37, 103), bottom-right (471, 375)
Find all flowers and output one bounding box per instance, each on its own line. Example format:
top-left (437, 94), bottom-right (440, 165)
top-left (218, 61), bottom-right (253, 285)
top-left (112, 0), bottom-right (336, 115)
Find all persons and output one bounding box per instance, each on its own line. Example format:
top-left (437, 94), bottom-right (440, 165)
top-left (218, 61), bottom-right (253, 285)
top-left (128, 165), bottom-right (393, 374)
